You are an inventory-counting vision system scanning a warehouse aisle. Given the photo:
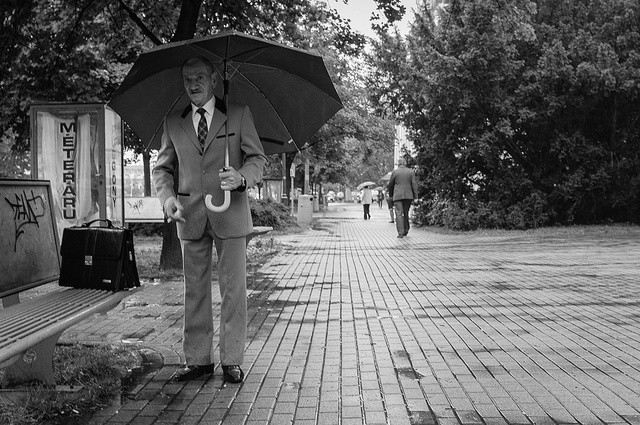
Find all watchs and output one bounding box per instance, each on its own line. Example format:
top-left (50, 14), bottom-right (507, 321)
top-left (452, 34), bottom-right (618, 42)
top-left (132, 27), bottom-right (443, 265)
top-left (241, 175), bottom-right (246, 187)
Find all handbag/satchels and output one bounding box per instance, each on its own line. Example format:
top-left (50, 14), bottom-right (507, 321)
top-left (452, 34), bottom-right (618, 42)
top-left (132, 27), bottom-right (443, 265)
top-left (58, 219), bottom-right (140, 292)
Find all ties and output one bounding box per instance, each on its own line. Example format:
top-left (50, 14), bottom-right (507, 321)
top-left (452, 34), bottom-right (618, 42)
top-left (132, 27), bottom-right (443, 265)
top-left (196, 107), bottom-right (208, 150)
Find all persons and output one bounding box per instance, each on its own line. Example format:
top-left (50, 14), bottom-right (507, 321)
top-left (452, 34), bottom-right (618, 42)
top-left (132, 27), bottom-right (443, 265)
top-left (377, 190), bottom-right (385, 208)
top-left (388, 157), bottom-right (419, 238)
top-left (386, 192), bottom-right (395, 223)
top-left (361, 186), bottom-right (372, 220)
top-left (151, 57), bottom-right (268, 383)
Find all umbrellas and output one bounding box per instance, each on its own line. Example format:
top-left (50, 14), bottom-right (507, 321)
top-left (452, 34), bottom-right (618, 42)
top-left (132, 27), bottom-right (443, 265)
top-left (357, 182), bottom-right (375, 190)
top-left (374, 186), bottom-right (386, 190)
top-left (106, 30), bottom-right (343, 213)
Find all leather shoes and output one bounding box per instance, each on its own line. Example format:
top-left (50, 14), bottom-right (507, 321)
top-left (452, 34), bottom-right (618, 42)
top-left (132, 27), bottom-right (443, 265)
top-left (178, 363), bottom-right (214, 380)
top-left (221, 365), bottom-right (244, 383)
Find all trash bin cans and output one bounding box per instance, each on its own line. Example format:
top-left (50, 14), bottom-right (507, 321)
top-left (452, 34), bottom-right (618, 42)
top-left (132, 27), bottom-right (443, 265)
top-left (297, 195), bottom-right (313, 226)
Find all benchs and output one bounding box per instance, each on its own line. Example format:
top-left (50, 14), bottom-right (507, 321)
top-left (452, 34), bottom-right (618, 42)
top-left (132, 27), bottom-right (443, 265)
top-left (215, 226), bottom-right (273, 264)
top-left (0, 179), bottom-right (144, 384)
top-left (112, 197), bottom-right (164, 229)
